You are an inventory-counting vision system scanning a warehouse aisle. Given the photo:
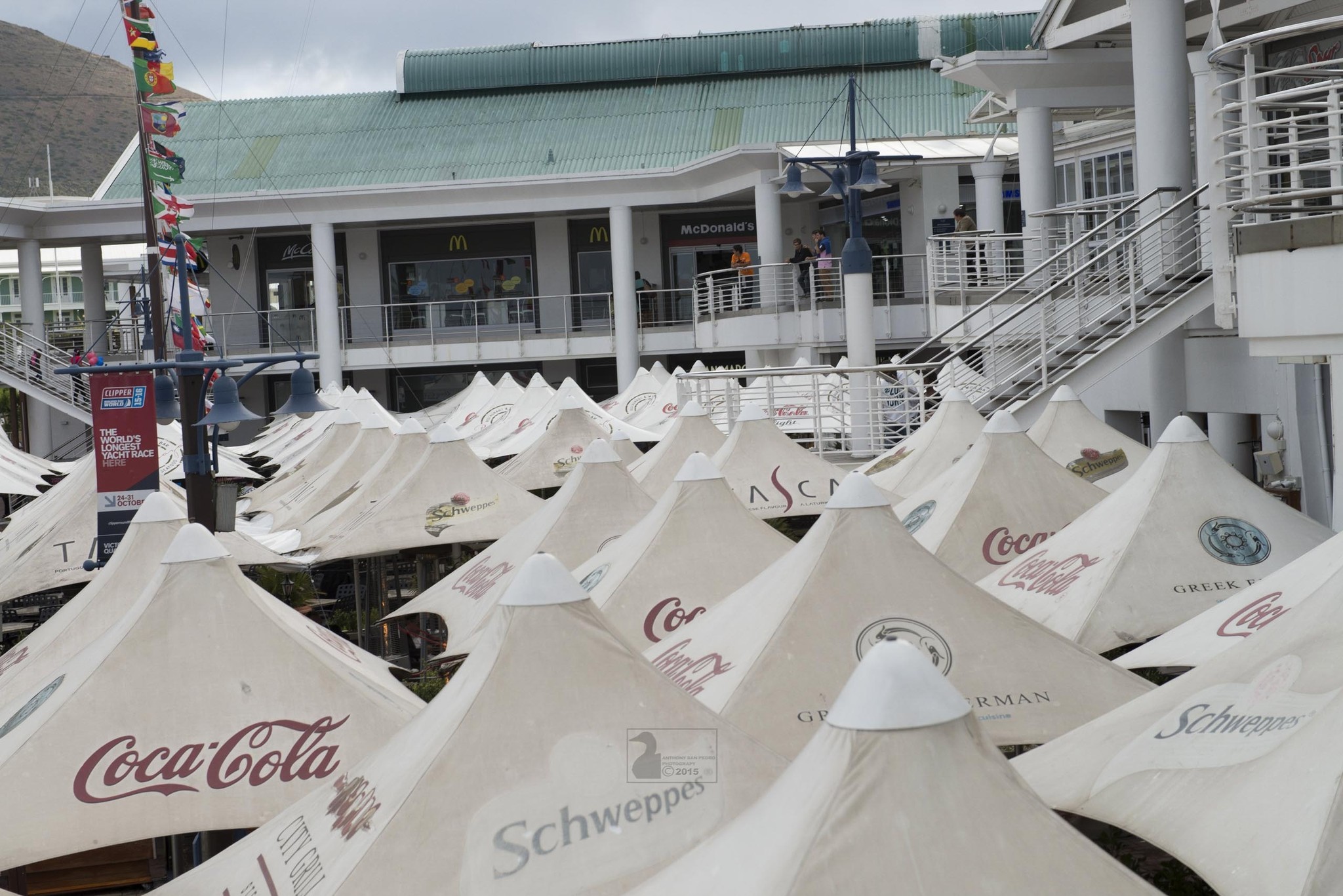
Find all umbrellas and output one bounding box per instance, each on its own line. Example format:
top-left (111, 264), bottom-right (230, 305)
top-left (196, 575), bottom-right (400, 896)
top-left (0, 355), bottom-right (1343, 896)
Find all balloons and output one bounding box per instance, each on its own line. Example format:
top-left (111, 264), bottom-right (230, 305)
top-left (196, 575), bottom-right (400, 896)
top-left (96, 356), bottom-right (104, 366)
top-left (87, 352), bottom-right (96, 359)
top-left (89, 357), bottom-right (98, 366)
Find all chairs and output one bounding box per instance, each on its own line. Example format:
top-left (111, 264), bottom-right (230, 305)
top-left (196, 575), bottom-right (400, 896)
top-left (407, 299), bottom-right (532, 327)
top-left (591, 297), bottom-right (606, 318)
top-left (247, 562), bottom-right (447, 670)
top-left (0, 592), bottom-right (64, 656)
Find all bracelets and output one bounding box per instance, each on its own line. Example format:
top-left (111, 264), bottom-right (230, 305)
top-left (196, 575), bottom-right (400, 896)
top-left (815, 242), bottom-right (818, 244)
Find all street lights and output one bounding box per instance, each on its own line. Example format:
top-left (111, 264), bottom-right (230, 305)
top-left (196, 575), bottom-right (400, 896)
top-left (771, 78), bottom-right (922, 460)
top-left (52, 232), bottom-right (339, 534)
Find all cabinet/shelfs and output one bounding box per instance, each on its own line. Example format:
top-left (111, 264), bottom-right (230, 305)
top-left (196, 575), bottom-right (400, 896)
top-left (272, 310), bottom-right (347, 347)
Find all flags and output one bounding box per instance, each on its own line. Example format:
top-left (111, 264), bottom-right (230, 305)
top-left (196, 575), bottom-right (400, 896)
top-left (120, 0), bottom-right (218, 390)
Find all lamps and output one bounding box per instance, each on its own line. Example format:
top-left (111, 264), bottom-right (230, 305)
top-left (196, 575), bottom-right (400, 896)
top-left (801, 225), bottom-right (806, 234)
top-left (640, 238), bottom-right (646, 244)
top-left (785, 228), bottom-right (792, 235)
top-left (226, 261), bottom-right (234, 268)
top-left (907, 204), bottom-right (914, 214)
top-left (938, 204), bottom-right (947, 214)
top-left (280, 574), bottom-right (295, 597)
top-left (360, 253), bottom-right (366, 259)
top-left (1270, 479), bottom-right (1297, 489)
top-left (1267, 421), bottom-right (1284, 441)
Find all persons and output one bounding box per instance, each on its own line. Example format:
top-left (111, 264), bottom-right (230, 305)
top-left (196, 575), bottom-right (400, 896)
top-left (803, 230), bottom-right (824, 302)
top-left (71, 350), bottom-right (90, 403)
top-left (29, 349), bottom-right (42, 383)
top-left (635, 271), bottom-right (651, 328)
top-left (785, 238), bottom-right (814, 298)
top-left (951, 208), bottom-right (988, 286)
top-left (731, 245), bottom-right (754, 309)
top-left (814, 231), bottom-right (835, 302)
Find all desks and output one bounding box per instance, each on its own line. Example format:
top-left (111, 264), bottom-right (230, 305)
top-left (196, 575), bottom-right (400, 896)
top-left (1262, 488), bottom-right (1301, 511)
top-left (387, 573), bottom-right (415, 583)
top-left (425, 305), bottom-right (446, 327)
top-left (388, 588), bottom-right (418, 599)
top-left (8, 592), bottom-right (64, 604)
top-left (3, 622), bottom-right (42, 633)
top-left (4, 606), bottom-right (60, 616)
top-left (487, 301), bottom-right (510, 325)
top-left (304, 598), bottom-right (341, 607)
